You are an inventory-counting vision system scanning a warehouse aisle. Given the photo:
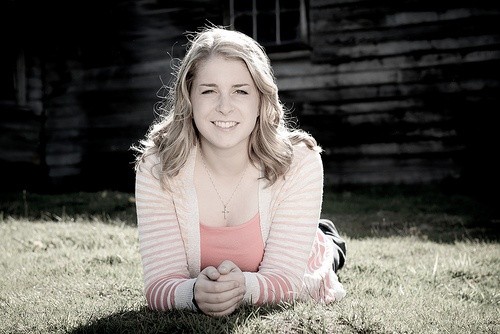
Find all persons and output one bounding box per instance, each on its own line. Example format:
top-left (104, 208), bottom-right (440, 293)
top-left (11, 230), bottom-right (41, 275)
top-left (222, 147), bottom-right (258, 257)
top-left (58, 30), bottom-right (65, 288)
top-left (124, 25), bottom-right (347, 317)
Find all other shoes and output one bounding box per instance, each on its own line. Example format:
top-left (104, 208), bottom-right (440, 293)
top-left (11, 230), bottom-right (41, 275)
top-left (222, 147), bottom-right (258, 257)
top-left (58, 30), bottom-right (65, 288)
top-left (319, 219), bottom-right (346, 269)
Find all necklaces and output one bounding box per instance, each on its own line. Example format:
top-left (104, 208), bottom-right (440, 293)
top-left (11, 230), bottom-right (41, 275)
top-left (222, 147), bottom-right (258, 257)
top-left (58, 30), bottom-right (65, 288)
top-left (199, 149), bottom-right (250, 219)
